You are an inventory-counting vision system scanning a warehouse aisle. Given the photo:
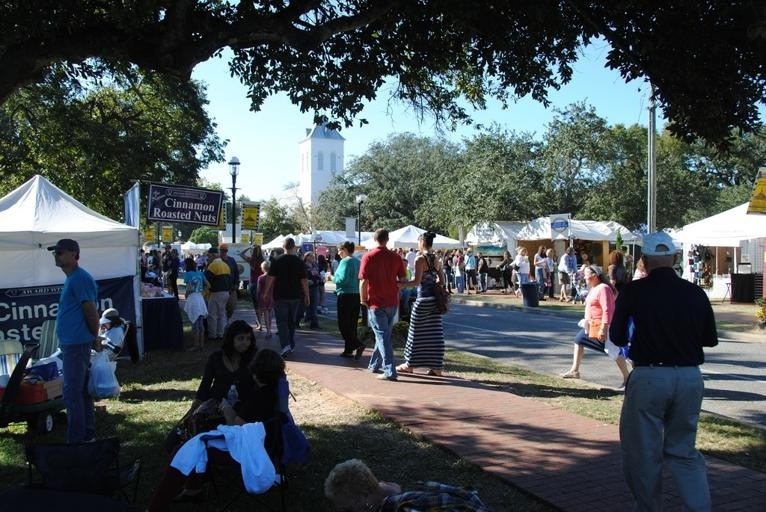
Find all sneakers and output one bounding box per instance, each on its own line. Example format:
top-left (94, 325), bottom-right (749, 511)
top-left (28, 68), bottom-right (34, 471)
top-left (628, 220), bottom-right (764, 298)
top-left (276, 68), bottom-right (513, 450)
top-left (355, 344), bottom-right (365, 360)
top-left (376, 373), bottom-right (396, 381)
top-left (368, 366), bottom-right (377, 372)
top-left (340, 352), bottom-right (352, 357)
top-left (563, 371), bottom-right (579, 378)
top-left (280, 345), bottom-right (295, 357)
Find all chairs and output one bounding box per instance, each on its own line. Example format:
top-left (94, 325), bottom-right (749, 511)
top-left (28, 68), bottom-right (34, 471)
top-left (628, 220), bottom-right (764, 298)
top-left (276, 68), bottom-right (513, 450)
top-left (201, 417), bottom-right (296, 509)
top-left (93, 311), bottom-right (130, 370)
top-left (0, 485), bottom-right (132, 512)
top-left (0, 336), bottom-right (27, 385)
top-left (23, 431), bottom-right (141, 505)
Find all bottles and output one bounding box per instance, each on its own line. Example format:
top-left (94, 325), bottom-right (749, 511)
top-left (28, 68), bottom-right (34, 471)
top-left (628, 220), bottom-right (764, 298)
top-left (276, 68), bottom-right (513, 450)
top-left (102, 340), bottom-right (120, 353)
top-left (227, 384), bottom-right (238, 403)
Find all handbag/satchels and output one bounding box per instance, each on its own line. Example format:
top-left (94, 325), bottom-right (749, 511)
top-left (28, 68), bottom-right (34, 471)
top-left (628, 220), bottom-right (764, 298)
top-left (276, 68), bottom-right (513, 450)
top-left (145, 272), bottom-right (155, 279)
top-left (589, 319), bottom-right (620, 361)
top-left (164, 428), bottom-right (181, 455)
top-left (281, 421), bottom-right (309, 464)
top-left (560, 272), bottom-right (571, 284)
top-left (60, 439), bottom-right (119, 471)
top-left (433, 284), bottom-right (452, 315)
top-left (502, 264), bottom-right (519, 271)
top-left (226, 291), bottom-right (237, 311)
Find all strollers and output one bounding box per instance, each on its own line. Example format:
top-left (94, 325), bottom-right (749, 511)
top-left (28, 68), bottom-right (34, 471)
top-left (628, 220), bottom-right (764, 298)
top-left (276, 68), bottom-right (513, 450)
top-left (571, 274), bottom-right (587, 304)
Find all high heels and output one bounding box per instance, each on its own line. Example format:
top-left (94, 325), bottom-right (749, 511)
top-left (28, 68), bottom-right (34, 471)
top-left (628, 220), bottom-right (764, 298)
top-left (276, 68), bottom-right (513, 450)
top-left (175, 482), bottom-right (209, 501)
top-left (269, 475), bottom-right (297, 495)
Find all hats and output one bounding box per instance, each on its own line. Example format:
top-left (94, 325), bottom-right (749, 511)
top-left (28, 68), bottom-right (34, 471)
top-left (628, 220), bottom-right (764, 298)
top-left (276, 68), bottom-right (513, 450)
top-left (208, 248), bottom-right (218, 253)
top-left (99, 308), bottom-right (120, 324)
top-left (641, 232), bottom-right (675, 256)
top-left (48, 239), bottom-right (80, 252)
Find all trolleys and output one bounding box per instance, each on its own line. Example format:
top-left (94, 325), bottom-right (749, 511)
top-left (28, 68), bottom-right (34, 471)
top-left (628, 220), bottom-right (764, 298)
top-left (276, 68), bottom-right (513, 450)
top-left (0, 338), bottom-right (71, 436)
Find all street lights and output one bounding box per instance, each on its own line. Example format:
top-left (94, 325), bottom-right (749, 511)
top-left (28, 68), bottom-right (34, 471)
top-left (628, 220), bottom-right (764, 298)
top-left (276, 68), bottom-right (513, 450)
top-left (226, 156), bottom-right (241, 244)
top-left (354, 193), bottom-right (369, 249)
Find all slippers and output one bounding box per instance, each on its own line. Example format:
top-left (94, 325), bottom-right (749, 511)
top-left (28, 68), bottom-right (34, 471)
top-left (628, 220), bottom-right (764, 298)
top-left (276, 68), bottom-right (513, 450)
top-left (428, 369), bottom-right (441, 376)
top-left (397, 364), bottom-right (413, 373)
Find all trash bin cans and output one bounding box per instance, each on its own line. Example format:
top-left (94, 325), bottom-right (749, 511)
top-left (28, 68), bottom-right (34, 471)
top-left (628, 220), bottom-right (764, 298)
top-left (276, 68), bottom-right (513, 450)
top-left (521, 283), bottom-right (540, 307)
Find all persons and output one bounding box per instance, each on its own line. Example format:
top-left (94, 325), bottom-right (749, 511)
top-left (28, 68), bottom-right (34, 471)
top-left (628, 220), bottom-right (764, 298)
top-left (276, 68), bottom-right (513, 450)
top-left (438, 248), bottom-right (488, 295)
top-left (325, 460), bottom-right (487, 512)
top-left (608, 232), bottom-right (718, 511)
top-left (92, 305), bottom-right (125, 356)
top-left (563, 251), bottom-right (647, 389)
top-left (496, 245), bottom-right (591, 305)
top-left (136, 243), bottom-right (240, 351)
top-left (240, 228), bottom-right (445, 382)
top-left (48, 235), bottom-right (98, 441)
top-left (674, 264), bottom-right (713, 287)
top-left (145, 321), bottom-right (289, 512)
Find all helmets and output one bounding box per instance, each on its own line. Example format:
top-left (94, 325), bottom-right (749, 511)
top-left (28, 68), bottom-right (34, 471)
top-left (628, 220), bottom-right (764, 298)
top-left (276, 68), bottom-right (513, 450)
top-left (220, 244), bottom-right (228, 251)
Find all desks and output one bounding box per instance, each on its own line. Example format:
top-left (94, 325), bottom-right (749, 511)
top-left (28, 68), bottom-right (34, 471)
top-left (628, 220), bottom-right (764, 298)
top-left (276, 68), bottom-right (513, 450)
top-left (140, 278), bottom-right (186, 355)
